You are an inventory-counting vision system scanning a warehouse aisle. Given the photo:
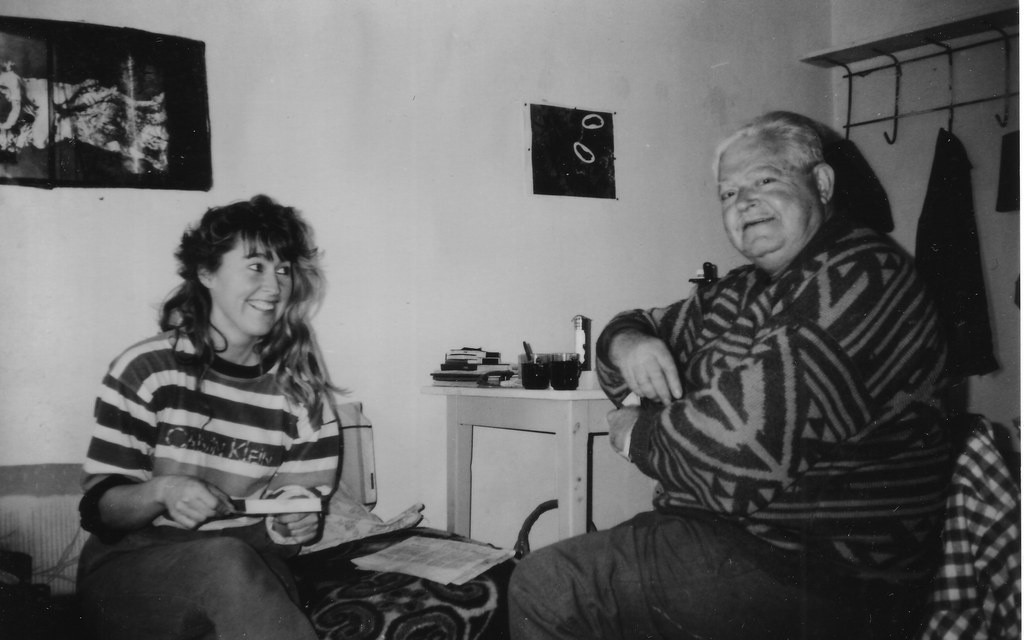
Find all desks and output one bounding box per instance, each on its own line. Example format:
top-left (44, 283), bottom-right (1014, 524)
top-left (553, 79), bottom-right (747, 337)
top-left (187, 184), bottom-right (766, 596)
top-left (425, 387), bottom-right (620, 541)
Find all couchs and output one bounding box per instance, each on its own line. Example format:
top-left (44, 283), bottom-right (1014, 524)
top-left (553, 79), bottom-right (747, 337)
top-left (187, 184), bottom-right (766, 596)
top-left (0, 403), bottom-right (519, 640)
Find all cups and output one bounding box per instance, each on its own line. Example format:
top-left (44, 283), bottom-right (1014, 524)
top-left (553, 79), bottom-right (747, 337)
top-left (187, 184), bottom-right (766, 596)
top-left (521, 353), bottom-right (550, 389)
top-left (550, 353), bottom-right (582, 390)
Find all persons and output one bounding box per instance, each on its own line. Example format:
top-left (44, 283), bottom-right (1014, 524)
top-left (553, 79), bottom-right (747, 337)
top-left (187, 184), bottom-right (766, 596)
top-left (73, 194), bottom-right (346, 640)
top-left (508, 109), bottom-right (958, 640)
